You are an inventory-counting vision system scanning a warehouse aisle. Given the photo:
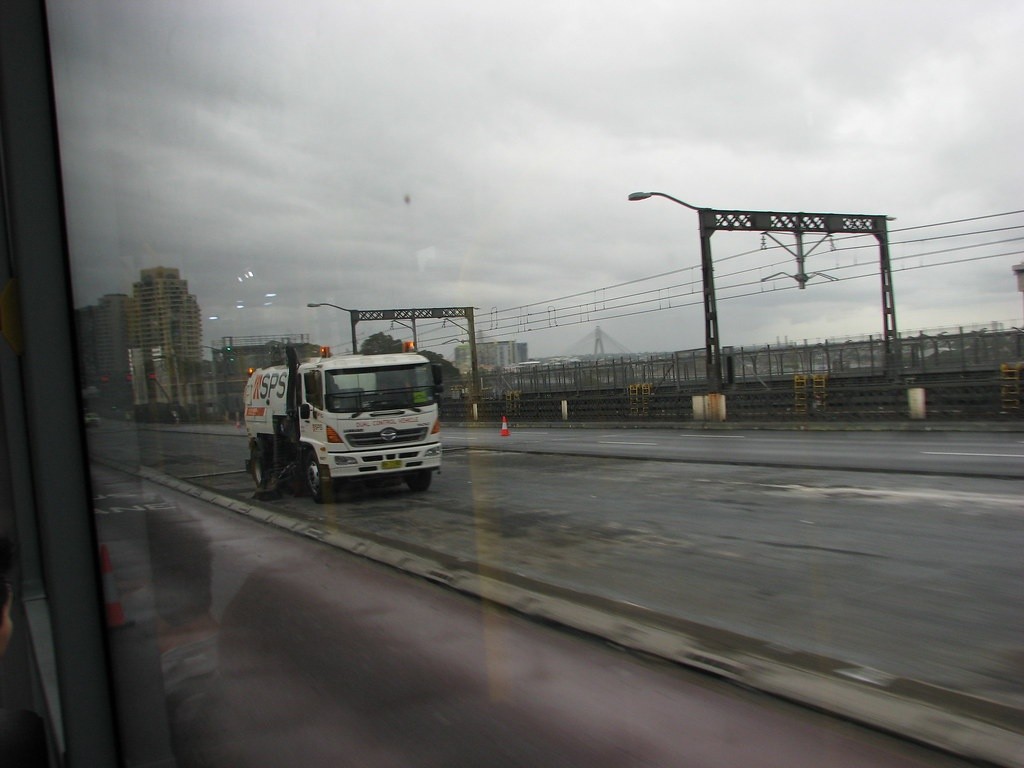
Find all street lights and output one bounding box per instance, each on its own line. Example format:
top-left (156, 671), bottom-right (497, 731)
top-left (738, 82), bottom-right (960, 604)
top-left (306, 302), bottom-right (357, 355)
top-left (628, 191), bottom-right (722, 392)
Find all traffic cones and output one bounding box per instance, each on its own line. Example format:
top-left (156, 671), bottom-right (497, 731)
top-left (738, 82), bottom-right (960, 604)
top-left (500, 416), bottom-right (510, 436)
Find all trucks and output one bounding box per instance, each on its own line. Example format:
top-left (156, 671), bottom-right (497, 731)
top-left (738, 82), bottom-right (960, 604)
top-left (242, 344), bottom-right (445, 503)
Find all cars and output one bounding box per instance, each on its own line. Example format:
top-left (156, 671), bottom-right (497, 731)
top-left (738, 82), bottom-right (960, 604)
top-left (85, 413), bottom-right (101, 426)
